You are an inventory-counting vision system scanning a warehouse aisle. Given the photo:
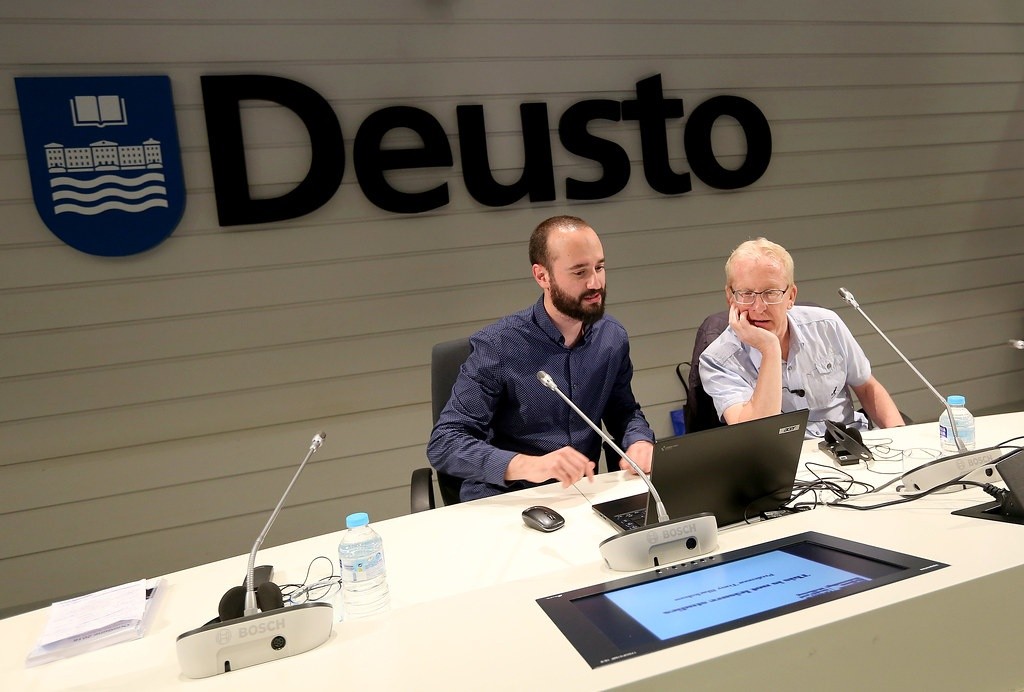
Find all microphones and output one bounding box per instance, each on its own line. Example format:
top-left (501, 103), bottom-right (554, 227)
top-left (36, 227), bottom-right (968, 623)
top-left (535, 369), bottom-right (719, 573)
top-left (791, 389), bottom-right (805, 397)
top-left (837, 287), bottom-right (1004, 496)
top-left (176, 431), bottom-right (328, 680)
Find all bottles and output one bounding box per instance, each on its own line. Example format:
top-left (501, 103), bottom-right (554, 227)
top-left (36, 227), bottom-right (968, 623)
top-left (339, 511), bottom-right (389, 625)
top-left (939, 395), bottom-right (978, 456)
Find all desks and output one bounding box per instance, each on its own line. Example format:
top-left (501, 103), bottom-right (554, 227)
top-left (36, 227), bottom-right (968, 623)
top-left (0, 412), bottom-right (1024, 692)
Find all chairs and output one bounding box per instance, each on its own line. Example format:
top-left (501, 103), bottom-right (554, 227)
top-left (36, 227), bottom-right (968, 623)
top-left (687, 303), bottom-right (915, 434)
top-left (411, 337), bottom-right (625, 514)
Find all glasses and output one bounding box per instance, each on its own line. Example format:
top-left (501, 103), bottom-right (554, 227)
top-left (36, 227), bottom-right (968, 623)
top-left (730, 284), bottom-right (790, 305)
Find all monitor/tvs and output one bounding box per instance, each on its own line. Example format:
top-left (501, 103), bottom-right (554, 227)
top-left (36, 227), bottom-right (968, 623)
top-left (569, 541), bottom-right (908, 650)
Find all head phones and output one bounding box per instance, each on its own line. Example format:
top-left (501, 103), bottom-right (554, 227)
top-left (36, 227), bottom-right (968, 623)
top-left (824, 419), bottom-right (873, 461)
top-left (204, 581), bottom-right (284, 625)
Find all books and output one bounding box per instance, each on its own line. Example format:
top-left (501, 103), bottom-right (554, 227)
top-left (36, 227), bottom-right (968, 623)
top-left (25, 578), bottom-right (166, 668)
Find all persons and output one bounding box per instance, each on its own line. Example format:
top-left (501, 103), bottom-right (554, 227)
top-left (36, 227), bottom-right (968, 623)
top-left (699, 237), bottom-right (906, 440)
top-left (426, 216), bottom-right (655, 503)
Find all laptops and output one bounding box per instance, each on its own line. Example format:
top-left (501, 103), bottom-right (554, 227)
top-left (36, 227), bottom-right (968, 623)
top-left (591, 409), bottom-right (811, 533)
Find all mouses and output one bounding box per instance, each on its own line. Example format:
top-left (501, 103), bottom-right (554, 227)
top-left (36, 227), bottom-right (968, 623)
top-left (522, 505), bottom-right (565, 533)
